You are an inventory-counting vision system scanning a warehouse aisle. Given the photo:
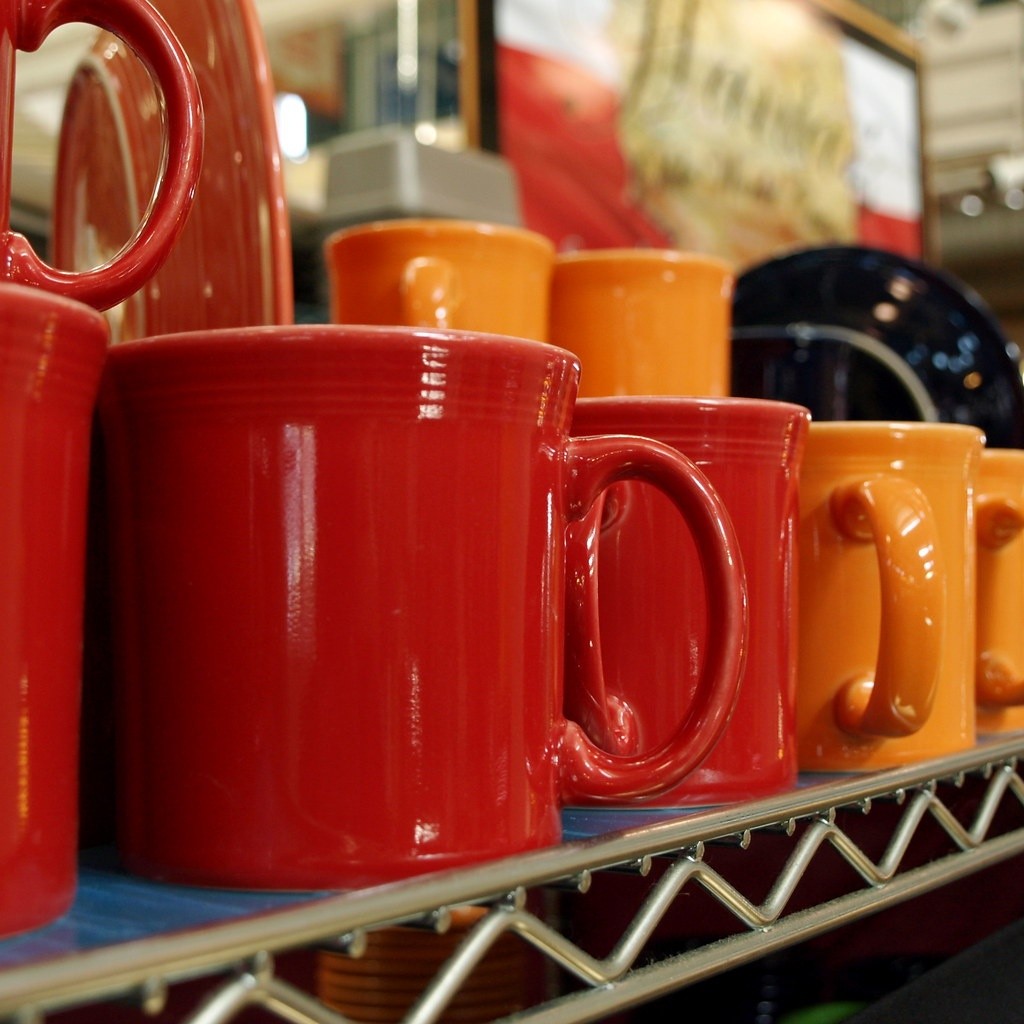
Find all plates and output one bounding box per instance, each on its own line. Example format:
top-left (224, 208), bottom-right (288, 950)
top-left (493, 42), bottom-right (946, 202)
top-left (731, 239), bottom-right (1024, 447)
top-left (53, 0), bottom-right (298, 342)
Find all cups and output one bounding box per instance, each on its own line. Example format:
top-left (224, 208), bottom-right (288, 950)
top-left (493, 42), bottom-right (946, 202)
top-left (1, 289), bottom-right (116, 940)
top-left (551, 247), bottom-right (734, 396)
top-left (100, 324), bottom-right (751, 895)
top-left (559, 393), bottom-right (813, 811)
top-left (797, 419), bottom-right (986, 774)
top-left (0, 1), bottom-right (200, 311)
top-left (973, 448), bottom-right (1024, 737)
top-left (324, 218), bottom-right (551, 344)
